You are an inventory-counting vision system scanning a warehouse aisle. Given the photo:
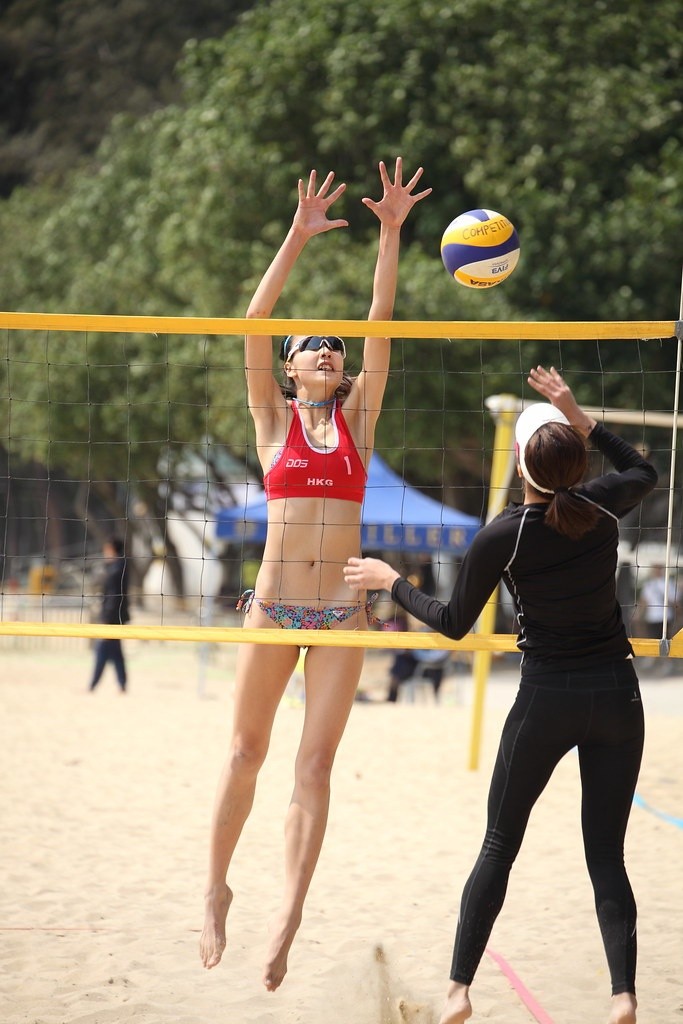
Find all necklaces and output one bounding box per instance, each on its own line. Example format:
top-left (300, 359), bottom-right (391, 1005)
top-left (293, 395), bottom-right (336, 406)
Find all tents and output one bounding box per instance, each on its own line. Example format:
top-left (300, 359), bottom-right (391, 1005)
top-left (196, 449), bottom-right (483, 697)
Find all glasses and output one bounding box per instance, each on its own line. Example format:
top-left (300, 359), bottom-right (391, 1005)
top-left (286, 336), bottom-right (346, 362)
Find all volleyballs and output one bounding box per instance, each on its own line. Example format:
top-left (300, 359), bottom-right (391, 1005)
top-left (439, 208), bottom-right (521, 289)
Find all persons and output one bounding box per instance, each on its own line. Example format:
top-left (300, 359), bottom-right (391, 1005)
top-left (615, 563), bottom-right (640, 638)
top-left (199, 156), bottom-right (432, 993)
top-left (367, 571), bottom-right (443, 702)
top-left (88, 538), bottom-right (130, 693)
top-left (343, 366), bottom-right (659, 1024)
top-left (635, 563), bottom-right (678, 677)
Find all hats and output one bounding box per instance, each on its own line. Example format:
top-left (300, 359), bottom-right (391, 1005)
top-left (515, 403), bottom-right (574, 494)
